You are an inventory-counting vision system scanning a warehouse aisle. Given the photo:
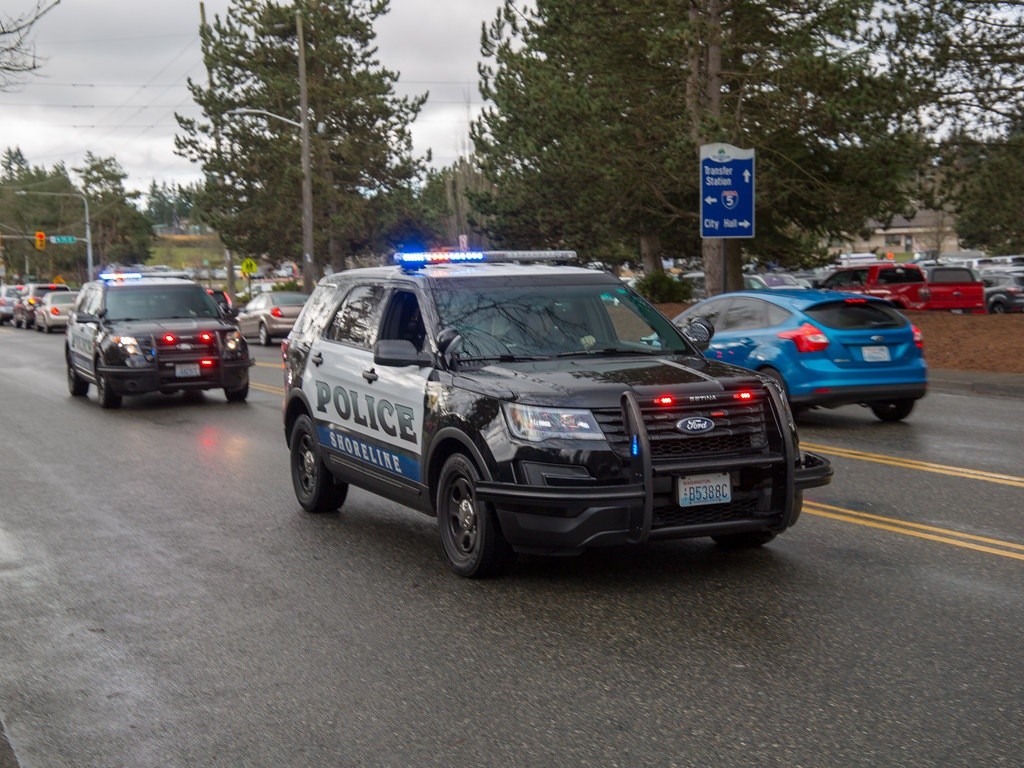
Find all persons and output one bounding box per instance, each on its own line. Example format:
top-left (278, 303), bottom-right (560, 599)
top-left (503, 303), bottom-right (597, 357)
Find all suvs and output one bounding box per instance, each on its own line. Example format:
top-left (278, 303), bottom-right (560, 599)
top-left (284, 249), bottom-right (833, 578)
top-left (66, 272), bottom-right (249, 410)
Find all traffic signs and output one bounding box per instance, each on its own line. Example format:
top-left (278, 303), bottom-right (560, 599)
top-left (700, 143), bottom-right (755, 238)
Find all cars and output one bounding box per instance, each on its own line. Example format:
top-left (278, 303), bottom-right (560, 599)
top-left (638, 288), bottom-right (929, 423)
top-left (13, 284), bottom-right (71, 328)
top-left (106, 238), bottom-right (340, 320)
top-left (33, 290), bottom-right (80, 332)
top-left (586, 224), bottom-right (1024, 322)
top-left (1, 284), bottom-right (24, 325)
top-left (236, 291), bottom-right (308, 344)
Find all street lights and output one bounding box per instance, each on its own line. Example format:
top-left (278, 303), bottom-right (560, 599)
top-left (229, 46), bottom-right (316, 293)
top-left (14, 190), bottom-right (94, 282)
